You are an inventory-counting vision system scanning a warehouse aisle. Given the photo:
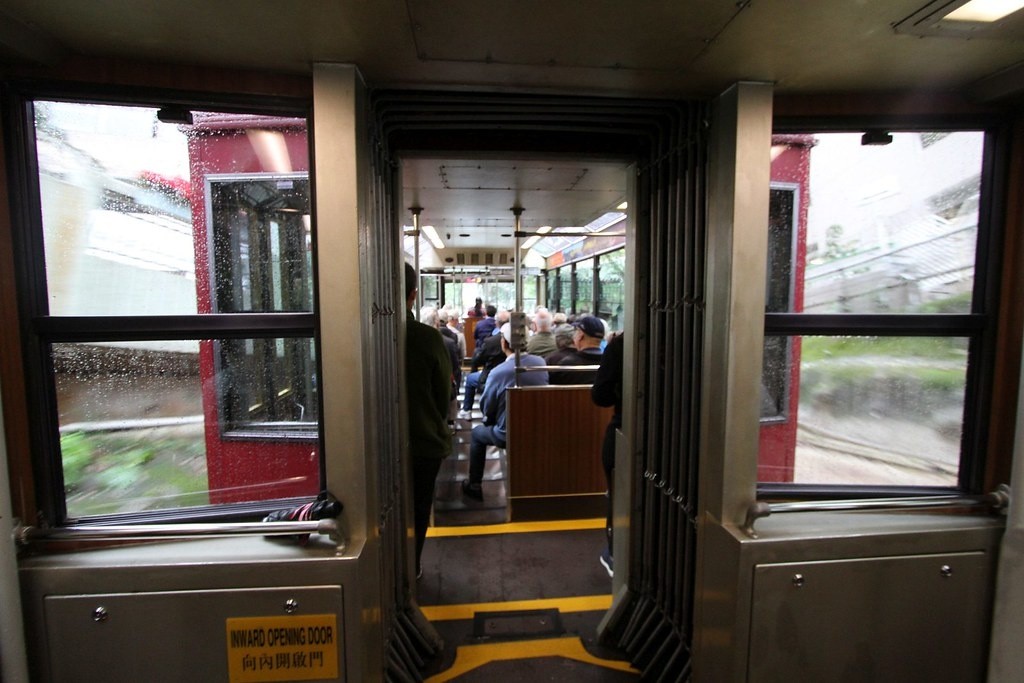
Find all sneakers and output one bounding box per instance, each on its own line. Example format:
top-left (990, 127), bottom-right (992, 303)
top-left (459, 409), bottom-right (473, 421)
top-left (445, 422), bottom-right (456, 435)
top-left (600, 546), bottom-right (615, 577)
top-left (463, 478), bottom-right (483, 500)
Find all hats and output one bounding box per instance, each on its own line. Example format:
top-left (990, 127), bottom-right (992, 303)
top-left (499, 321), bottom-right (530, 348)
top-left (571, 317), bottom-right (604, 339)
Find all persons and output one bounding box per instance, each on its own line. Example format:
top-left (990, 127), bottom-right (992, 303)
top-left (417, 306), bottom-right (576, 426)
top-left (462, 324), bottom-right (548, 499)
top-left (592, 330), bottom-right (624, 579)
top-left (468, 298), bottom-right (486, 317)
top-left (405, 264), bottom-right (453, 578)
top-left (551, 318), bottom-right (604, 384)
